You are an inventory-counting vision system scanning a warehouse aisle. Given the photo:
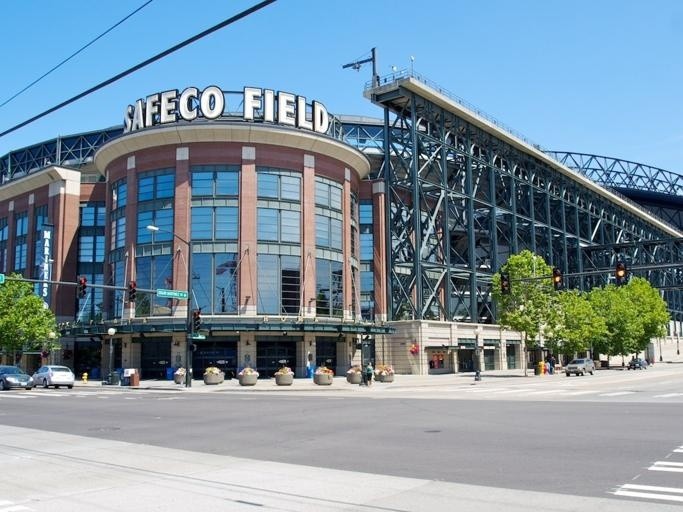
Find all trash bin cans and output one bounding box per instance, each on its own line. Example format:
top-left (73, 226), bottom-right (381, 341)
top-left (307, 367), bottom-right (314, 378)
top-left (166, 367), bottom-right (174, 380)
top-left (535, 365), bottom-right (542, 375)
top-left (91, 367), bottom-right (100, 379)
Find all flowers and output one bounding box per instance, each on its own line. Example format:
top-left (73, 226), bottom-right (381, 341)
top-left (409, 344), bottom-right (419, 354)
top-left (174, 367), bottom-right (398, 375)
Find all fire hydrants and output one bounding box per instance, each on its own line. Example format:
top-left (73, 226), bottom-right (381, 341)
top-left (82, 372), bottom-right (88, 384)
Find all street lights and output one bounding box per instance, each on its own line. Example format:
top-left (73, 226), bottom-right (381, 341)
top-left (145, 225), bottom-right (192, 388)
top-left (108, 328), bottom-right (115, 371)
top-left (473, 328), bottom-right (481, 381)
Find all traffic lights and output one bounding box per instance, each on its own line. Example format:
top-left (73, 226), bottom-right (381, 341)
top-left (616, 261), bottom-right (626, 285)
top-left (501, 272), bottom-right (510, 294)
top-left (128, 282), bottom-right (136, 302)
top-left (192, 307), bottom-right (201, 333)
top-left (553, 268), bottom-right (563, 290)
top-left (77, 276), bottom-right (87, 299)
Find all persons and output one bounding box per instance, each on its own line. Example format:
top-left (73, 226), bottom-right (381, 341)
top-left (544, 356), bottom-right (556, 375)
top-left (367, 362), bottom-right (373, 386)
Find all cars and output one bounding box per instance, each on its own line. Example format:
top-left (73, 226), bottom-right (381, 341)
top-left (565, 358), bottom-right (595, 376)
top-left (0, 365), bottom-right (74, 391)
top-left (627, 358), bottom-right (648, 369)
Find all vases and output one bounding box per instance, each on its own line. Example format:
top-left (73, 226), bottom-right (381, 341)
top-left (176, 372), bottom-right (394, 385)
